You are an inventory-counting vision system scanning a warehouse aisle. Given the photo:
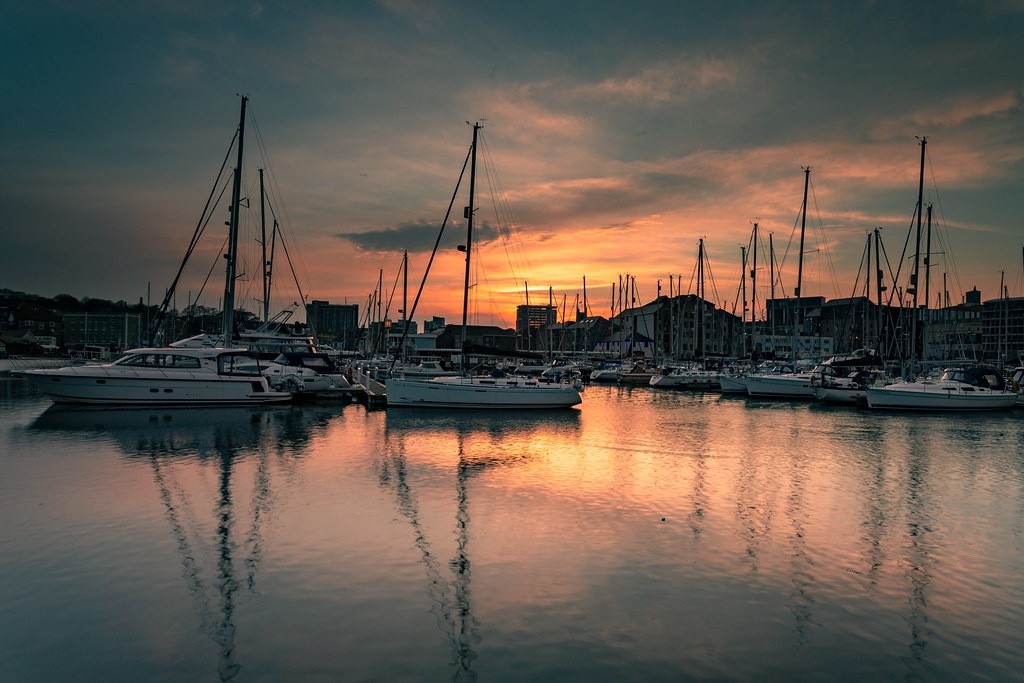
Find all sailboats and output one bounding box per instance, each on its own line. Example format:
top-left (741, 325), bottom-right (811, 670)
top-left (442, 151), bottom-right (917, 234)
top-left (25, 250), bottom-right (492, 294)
top-left (9, 92), bottom-right (1024, 410)
